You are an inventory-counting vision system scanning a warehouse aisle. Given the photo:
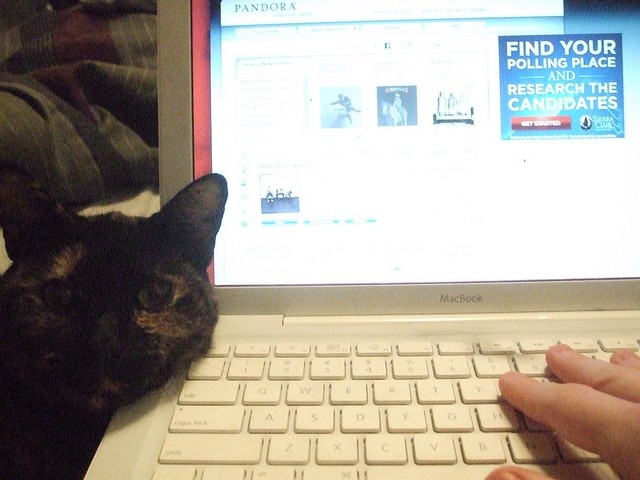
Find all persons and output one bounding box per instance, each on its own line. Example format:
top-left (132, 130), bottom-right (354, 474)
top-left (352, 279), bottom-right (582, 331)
top-left (486, 340), bottom-right (640, 480)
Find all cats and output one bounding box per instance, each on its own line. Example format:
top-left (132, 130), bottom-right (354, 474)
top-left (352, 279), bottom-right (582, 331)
top-left (0, 162), bottom-right (228, 480)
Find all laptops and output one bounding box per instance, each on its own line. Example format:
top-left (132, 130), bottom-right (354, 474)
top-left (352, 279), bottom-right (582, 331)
top-left (83, 1), bottom-right (639, 480)
